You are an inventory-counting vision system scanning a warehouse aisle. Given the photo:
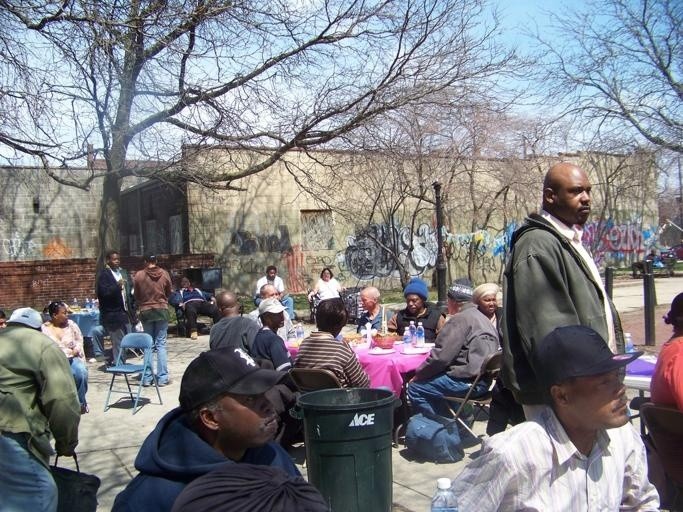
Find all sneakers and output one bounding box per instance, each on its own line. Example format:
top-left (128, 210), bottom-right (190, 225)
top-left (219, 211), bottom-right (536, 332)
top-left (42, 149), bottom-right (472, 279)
top-left (105, 359), bottom-right (131, 376)
top-left (142, 378), bottom-right (170, 387)
top-left (81, 402), bottom-right (88, 414)
top-left (189, 328), bottom-right (198, 340)
top-left (88, 354), bottom-right (104, 363)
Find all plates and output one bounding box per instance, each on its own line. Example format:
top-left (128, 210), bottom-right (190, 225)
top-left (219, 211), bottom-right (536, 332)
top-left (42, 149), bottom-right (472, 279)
top-left (420, 343), bottom-right (435, 348)
top-left (400, 348), bottom-right (433, 353)
top-left (369, 349), bottom-right (394, 355)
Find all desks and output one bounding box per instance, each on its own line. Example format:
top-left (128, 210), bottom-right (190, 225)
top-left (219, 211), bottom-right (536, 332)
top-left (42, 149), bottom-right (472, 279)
top-left (621, 355), bottom-right (657, 434)
top-left (43, 308), bottom-right (100, 337)
top-left (285, 333), bottom-right (435, 449)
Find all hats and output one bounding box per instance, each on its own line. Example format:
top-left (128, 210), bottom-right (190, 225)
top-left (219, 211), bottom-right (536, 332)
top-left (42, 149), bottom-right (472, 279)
top-left (178, 345), bottom-right (289, 412)
top-left (257, 296), bottom-right (286, 318)
top-left (404, 277), bottom-right (430, 304)
top-left (144, 251), bottom-right (157, 261)
top-left (5, 306), bottom-right (44, 330)
top-left (534, 326), bottom-right (642, 383)
top-left (447, 276), bottom-right (474, 303)
top-left (474, 282), bottom-right (500, 304)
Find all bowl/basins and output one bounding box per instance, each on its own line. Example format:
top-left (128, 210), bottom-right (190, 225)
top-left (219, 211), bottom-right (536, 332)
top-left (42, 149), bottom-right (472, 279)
top-left (373, 332), bottom-right (398, 348)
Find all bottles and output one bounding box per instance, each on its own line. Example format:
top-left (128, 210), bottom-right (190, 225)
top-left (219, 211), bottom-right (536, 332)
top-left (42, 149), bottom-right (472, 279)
top-left (296, 322), bottom-right (305, 337)
top-left (82, 298), bottom-right (97, 309)
top-left (429, 477), bottom-right (459, 511)
top-left (72, 297), bottom-right (78, 307)
top-left (403, 320), bottom-right (426, 349)
top-left (624, 331), bottom-right (634, 354)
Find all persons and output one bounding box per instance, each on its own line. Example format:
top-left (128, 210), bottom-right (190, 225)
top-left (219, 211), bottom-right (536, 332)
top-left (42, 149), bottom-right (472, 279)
top-left (650, 294), bottom-right (683, 413)
top-left (646, 249), bottom-right (658, 260)
top-left (485, 161), bottom-right (626, 435)
top-left (450, 324), bottom-right (661, 512)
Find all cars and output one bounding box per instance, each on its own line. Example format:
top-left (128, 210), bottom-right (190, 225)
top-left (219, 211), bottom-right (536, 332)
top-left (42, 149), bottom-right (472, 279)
top-left (659, 243), bottom-right (682, 261)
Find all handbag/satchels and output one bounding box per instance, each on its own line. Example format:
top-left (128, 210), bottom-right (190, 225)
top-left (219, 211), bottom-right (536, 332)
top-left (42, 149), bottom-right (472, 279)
top-left (402, 412), bottom-right (465, 464)
top-left (132, 319), bottom-right (144, 333)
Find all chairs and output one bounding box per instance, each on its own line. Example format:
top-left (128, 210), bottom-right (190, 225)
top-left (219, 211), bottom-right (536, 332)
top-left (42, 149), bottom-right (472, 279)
top-left (308, 290), bottom-right (348, 327)
top-left (639, 402), bottom-right (681, 512)
top-left (288, 369), bottom-right (344, 395)
top-left (441, 352), bottom-right (503, 443)
top-left (104, 333), bottom-right (163, 415)
top-left (175, 289), bottom-right (188, 338)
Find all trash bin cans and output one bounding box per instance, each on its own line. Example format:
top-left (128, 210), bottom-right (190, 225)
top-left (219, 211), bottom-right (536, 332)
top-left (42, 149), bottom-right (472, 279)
top-left (288, 387), bottom-right (402, 512)
